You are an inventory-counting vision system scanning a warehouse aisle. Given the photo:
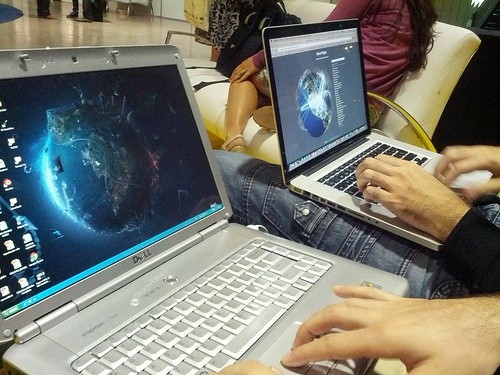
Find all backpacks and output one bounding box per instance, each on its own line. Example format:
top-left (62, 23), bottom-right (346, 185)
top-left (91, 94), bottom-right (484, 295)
top-left (215, 0), bottom-right (301, 79)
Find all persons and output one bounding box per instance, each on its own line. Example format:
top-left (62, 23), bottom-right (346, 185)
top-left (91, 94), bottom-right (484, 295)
top-left (217, 0), bottom-right (439, 153)
top-left (210, 0), bottom-right (254, 61)
top-left (204, 283), bottom-right (500, 375)
top-left (36, 0), bottom-right (108, 22)
top-left (214, 143), bottom-right (500, 296)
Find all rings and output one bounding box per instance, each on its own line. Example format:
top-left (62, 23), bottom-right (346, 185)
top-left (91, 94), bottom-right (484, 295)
top-left (240, 69), bottom-right (245, 74)
top-left (448, 163), bottom-right (458, 171)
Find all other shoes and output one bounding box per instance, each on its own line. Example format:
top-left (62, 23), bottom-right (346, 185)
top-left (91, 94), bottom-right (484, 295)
top-left (74, 17), bottom-right (91, 23)
top-left (38, 15), bottom-right (58, 19)
top-left (66, 13), bottom-right (78, 19)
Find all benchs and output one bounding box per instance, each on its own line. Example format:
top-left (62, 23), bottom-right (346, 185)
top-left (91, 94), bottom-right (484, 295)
top-left (163, 0), bottom-right (482, 165)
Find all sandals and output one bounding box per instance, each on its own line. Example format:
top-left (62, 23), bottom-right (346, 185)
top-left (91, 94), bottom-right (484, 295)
top-left (221, 135), bottom-right (246, 153)
top-left (253, 106), bottom-right (276, 133)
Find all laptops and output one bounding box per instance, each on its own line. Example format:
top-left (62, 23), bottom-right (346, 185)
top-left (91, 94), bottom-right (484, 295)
top-left (262, 18), bottom-right (493, 252)
top-left (1, 44), bottom-right (412, 375)
